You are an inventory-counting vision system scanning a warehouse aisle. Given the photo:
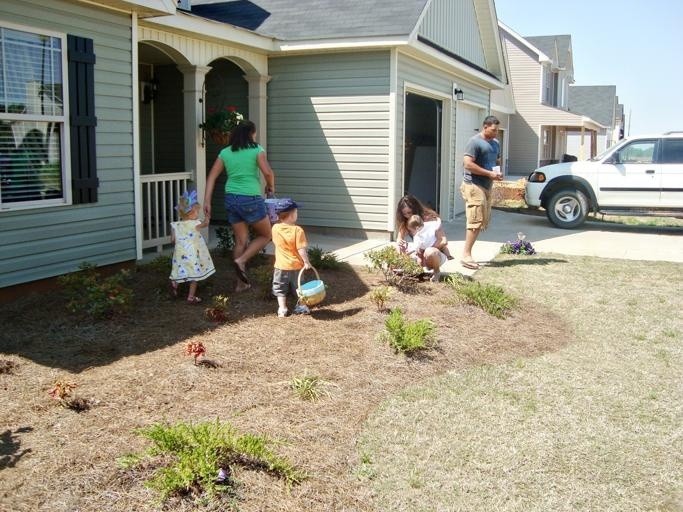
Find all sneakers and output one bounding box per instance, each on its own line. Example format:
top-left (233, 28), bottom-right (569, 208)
top-left (187, 296), bottom-right (202, 302)
top-left (461, 260), bottom-right (478, 269)
top-left (168, 280), bottom-right (179, 298)
top-left (293, 305), bottom-right (311, 315)
top-left (277, 306), bottom-right (292, 318)
top-left (429, 270), bottom-right (441, 282)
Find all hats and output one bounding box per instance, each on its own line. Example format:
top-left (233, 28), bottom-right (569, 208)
top-left (274, 197), bottom-right (304, 213)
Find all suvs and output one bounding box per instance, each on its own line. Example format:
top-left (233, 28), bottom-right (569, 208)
top-left (524, 130), bottom-right (682, 229)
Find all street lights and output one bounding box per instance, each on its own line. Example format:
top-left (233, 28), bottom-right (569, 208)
top-left (39, 34), bottom-right (49, 114)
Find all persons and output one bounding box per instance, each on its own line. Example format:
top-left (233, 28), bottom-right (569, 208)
top-left (459, 117), bottom-right (503, 269)
top-left (396, 194), bottom-right (446, 282)
top-left (407, 216), bottom-right (455, 261)
top-left (167, 189), bottom-right (215, 302)
top-left (203, 121), bottom-right (275, 291)
top-left (269, 199), bottom-right (309, 317)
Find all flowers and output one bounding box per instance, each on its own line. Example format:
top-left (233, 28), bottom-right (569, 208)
top-left (198, 104), bottom-right (243, 132)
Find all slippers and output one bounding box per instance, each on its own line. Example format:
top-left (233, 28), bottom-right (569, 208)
top-left (232, 260), bottom-right (249, 284)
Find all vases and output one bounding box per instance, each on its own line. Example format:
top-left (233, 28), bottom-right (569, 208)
top-left (210, 128), bottom-right (236, 145)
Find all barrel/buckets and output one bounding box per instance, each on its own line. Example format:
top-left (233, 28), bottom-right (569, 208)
top-left (264, 198), bottom-right (292, 226)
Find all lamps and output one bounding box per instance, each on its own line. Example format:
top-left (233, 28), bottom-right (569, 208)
top-left (452, 83), bottom-right (463, 102)
